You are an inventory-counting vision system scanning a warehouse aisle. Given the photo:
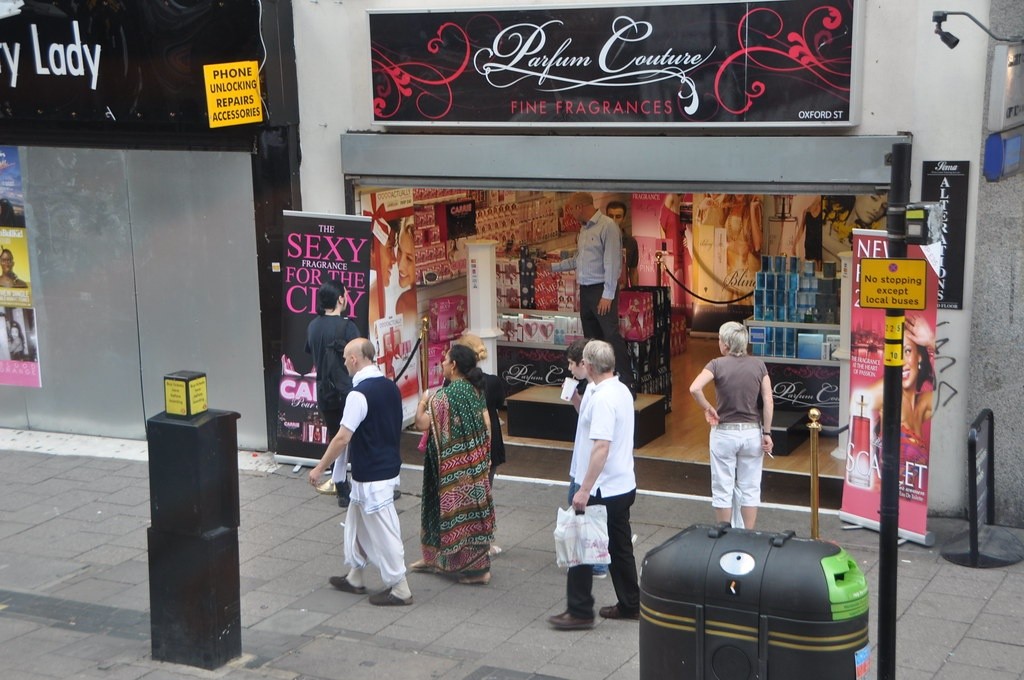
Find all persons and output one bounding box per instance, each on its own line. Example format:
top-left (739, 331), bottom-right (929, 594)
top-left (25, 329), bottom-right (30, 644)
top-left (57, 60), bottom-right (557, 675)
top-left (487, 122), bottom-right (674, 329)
top-left (660, 191), bottom-right (822, 306)
top-left (871, 313), bottom-right (934, 443)
top-left (456, 299), bottom-right (466, 332)
top-left (0, 248), bottom-right (27, 289)
top-left (822, 194), bottom-right (887, 242)
top-left (690, 322), bottom-right (774, 530)
top-left (547, 338), bottom-right (644, 631)
top-left (448, 239), bottom-right (459, 259)
top-left (409, 334), bottom-right (507, 585)
top-left (530, 192), bottom-right (640, 403)
top-left (308, 338), bottom-right (414, 606)
top-left (8, 323), bottom-right (25, 361)
top-left (304, 282), bottom-right (402, 508)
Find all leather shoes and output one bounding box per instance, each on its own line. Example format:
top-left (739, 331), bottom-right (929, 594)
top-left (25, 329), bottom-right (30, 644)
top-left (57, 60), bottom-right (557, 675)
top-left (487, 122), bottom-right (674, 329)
top-left (549, 610), bottom-right (594, 627)
top-left (599, 605), bottom-right (640, 619)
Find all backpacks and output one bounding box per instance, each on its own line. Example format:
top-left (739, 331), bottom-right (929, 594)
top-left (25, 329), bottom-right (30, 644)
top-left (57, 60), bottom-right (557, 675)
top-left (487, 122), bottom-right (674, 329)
top-left (321, 319), bottom-right (354, 403)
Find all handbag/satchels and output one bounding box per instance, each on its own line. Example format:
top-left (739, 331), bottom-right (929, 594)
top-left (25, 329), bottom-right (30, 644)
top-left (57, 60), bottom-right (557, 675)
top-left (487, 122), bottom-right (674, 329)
top-left (731, 486), bottom-right (746, 529)
top-left (554, 504), bottom-right (612, 568)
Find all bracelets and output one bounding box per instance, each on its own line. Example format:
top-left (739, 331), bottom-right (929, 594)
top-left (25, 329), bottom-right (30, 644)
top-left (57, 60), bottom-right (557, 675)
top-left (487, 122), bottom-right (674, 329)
top-left (417, 400), bottom-right (427, 408)
top-left (703, 404), bottom-right (712, 412)
top-left (762, 431), bottom-right (772, 436)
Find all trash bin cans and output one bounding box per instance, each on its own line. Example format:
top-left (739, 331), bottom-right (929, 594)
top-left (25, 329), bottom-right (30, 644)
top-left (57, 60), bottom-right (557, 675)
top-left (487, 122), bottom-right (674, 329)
top-left (640, 522), bottom-right (870, 680)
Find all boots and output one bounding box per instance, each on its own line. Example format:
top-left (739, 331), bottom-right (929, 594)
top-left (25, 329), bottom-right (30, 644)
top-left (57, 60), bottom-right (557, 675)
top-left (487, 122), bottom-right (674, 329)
top-left (394, 490), bottom-right (401, 500)
top-left (335, 481), bottom-right (350, 507)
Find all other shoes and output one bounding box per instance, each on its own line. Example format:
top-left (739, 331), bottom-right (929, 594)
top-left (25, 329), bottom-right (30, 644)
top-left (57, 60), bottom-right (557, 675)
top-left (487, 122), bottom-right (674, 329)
top-left (369, 587), bottom-right (412, 606)
top-left (410, 559), bottom-right (426, 568)
top-left (329, 573), bottom-right (367, 594)
top-left (458, 571), bottom-right (491, 585)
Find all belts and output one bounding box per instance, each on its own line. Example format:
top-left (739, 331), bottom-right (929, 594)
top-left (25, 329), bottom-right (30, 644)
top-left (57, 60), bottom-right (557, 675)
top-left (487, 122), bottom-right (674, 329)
top-left (717, 423), bottom-right (760, 430)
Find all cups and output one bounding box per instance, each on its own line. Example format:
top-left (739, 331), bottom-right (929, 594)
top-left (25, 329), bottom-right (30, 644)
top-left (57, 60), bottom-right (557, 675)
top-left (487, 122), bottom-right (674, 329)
top-left (560, 377), bottom-right (579, 401)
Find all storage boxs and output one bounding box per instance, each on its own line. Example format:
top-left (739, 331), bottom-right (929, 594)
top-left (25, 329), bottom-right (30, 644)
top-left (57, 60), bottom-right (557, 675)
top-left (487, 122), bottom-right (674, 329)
top-left (413, 187), bottom-right (686, 414)
top-left (751, 255), bottom-right (840, 360)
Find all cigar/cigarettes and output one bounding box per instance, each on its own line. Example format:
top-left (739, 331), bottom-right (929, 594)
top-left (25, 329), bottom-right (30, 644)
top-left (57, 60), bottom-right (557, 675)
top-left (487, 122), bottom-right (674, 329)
top-left (767, 452), bottom-right (774, 460)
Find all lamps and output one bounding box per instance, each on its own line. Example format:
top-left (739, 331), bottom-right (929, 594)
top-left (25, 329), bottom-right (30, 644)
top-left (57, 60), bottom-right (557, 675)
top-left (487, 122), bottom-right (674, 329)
top-left (932, 11), bottom-right (1024, 50)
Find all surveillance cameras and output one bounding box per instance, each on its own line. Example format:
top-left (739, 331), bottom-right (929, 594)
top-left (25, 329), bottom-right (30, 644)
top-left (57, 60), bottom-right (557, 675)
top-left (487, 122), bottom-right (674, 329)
top-left (935, 28), bottom-right (960, 48)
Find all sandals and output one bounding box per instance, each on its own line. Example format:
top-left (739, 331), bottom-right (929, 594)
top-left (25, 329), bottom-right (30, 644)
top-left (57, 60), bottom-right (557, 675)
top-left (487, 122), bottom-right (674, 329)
top-left (487, 546), bottom-right (503, 555)
